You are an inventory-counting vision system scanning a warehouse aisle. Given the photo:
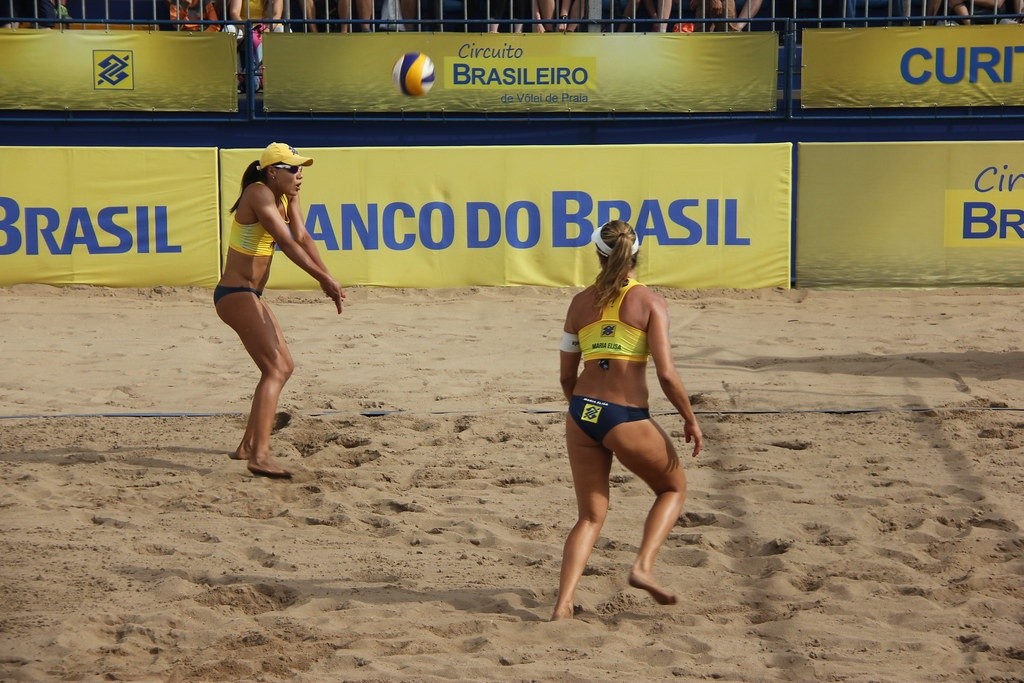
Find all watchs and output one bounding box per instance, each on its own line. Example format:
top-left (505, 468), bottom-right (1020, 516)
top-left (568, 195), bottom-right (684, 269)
top-left (559, 16), bottom-right (568, 20)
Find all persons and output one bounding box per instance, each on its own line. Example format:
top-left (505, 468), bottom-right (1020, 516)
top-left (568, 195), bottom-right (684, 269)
top-left (222, 0), bottom-right (292, 94)
top-left (489, 0), bottom-right (765, 34)
top-left (169, 0), bottom-right (221, 32)
top-left (0, 0), bottom-right (68, 30)
top-left (550, 220), bottom-right (703, 621)
top-left (300, 0), bottom-right (419, 33)
top-left (845, 0), bottom-right (1024, 26)
top-left (213, 142), bottom-right (345, 479)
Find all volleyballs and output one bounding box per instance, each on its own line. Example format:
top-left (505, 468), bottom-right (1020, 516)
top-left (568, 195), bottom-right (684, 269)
top-left (391, 52), bottom-right (435, 97)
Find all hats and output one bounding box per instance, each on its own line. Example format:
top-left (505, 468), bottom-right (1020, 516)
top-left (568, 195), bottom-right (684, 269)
top-left (591, 221), bottom-right (639, 256)
top-left (257, 142), bottom-right (313, 171)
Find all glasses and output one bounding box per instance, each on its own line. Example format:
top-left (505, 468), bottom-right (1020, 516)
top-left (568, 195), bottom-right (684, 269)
top-left (273, 165), bottom-right (303, 174)
